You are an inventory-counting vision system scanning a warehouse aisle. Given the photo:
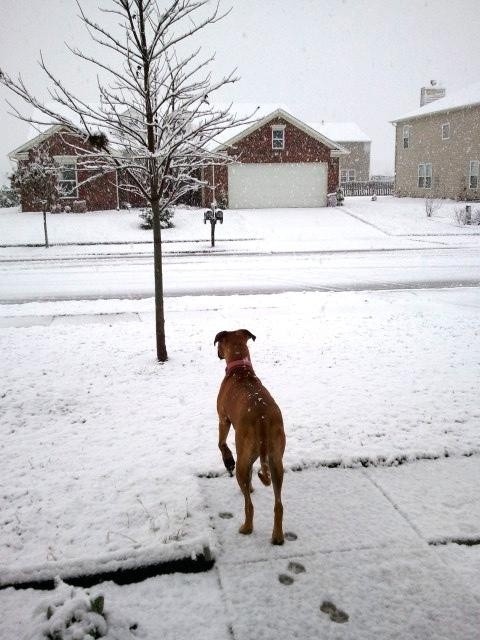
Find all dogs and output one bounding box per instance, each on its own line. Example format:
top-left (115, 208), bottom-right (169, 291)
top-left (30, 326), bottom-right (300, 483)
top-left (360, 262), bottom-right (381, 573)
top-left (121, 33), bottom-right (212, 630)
top-left (213, 328), bottom-right (287, 546)
